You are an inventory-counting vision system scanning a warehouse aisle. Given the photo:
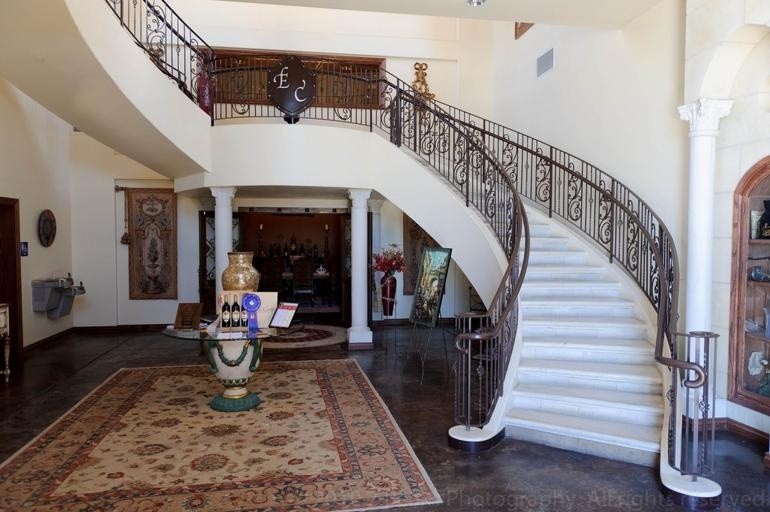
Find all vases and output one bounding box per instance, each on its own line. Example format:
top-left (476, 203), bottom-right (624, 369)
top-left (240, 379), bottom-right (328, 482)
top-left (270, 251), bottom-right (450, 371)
top-left (380, 274), bottom-right (397, 316)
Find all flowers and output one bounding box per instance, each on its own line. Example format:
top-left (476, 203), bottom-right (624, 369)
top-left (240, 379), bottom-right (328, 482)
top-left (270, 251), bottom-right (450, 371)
top-left (365, 242), bottom-right (408, 275)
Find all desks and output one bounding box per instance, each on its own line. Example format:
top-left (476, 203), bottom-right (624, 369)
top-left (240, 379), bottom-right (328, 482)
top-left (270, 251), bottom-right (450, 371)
top-left (162, 322), bottom-right (303, 414)
top-left (280, 275), bottom-right (333, 308)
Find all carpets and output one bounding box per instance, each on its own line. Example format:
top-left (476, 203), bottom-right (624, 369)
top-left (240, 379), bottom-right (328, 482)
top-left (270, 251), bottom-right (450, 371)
top-left (1, 364), bottom-right (454, 512)
top-left (262, 323), bottom-right (347, 350)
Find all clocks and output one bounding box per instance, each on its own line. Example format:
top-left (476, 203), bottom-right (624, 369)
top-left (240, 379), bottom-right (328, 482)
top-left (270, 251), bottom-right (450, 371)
top-left (36, 210), bottom-right (57, 247)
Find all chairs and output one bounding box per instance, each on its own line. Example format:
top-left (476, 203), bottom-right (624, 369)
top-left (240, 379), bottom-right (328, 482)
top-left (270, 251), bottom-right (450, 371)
top-left (261, 257), bottom-right (316, 307)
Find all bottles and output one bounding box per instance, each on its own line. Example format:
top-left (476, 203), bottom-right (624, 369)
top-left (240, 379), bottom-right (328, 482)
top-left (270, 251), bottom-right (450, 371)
top-left (222, 294), bottom-right (249, 326)
top-left (269, 237), bottom-right (318, 257)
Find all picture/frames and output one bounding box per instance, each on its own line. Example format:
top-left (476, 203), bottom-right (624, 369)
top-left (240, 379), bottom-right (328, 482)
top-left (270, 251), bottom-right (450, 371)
top-left (408, 247), bottom-right (452, 330)
